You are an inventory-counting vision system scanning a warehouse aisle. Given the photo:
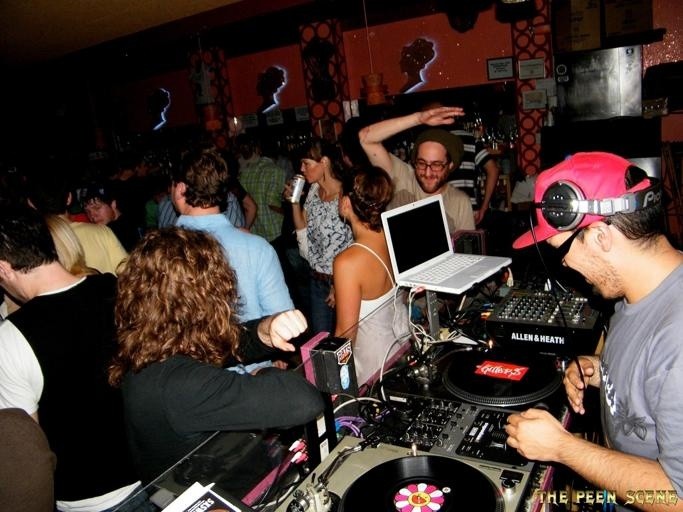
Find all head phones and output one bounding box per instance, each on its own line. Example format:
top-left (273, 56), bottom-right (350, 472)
top-left (541, 177), bottom-right (663, 231)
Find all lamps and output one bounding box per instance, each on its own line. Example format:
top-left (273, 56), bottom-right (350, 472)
top-left (360, 0), bottom-right (387, 107)
top-left (191, 28), bottom-right (225, 134)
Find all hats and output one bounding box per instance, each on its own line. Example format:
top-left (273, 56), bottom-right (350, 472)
top-left (410, 130), bottom-right (465, 173)
top-left (512, 151), bottom-right (652, 249)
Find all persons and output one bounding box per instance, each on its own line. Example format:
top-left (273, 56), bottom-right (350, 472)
top-left (254, 64), bottom-right (287, 113)
top-left (399, 38), bottom-right (436, 94)
top-left (144, 88), bottom-right (170, 131)
top-left (505, 152), bottom-right (681, 511)
top-left (109, 224), bottom-right (325, 512)
top-left (0, 206), bottom-right (147, 510)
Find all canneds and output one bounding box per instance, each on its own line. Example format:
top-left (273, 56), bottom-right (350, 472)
top-left (286, 173), bottom-right (305, 205)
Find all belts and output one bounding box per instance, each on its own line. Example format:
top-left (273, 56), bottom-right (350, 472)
top-left (310, 271), bottom-right (334, 281)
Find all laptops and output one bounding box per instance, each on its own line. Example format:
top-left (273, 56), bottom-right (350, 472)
top-left (380, 194), bottom-right (513, 295)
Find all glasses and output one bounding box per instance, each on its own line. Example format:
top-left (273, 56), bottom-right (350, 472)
top-left (414, 161), bottom-right (451, 170)
